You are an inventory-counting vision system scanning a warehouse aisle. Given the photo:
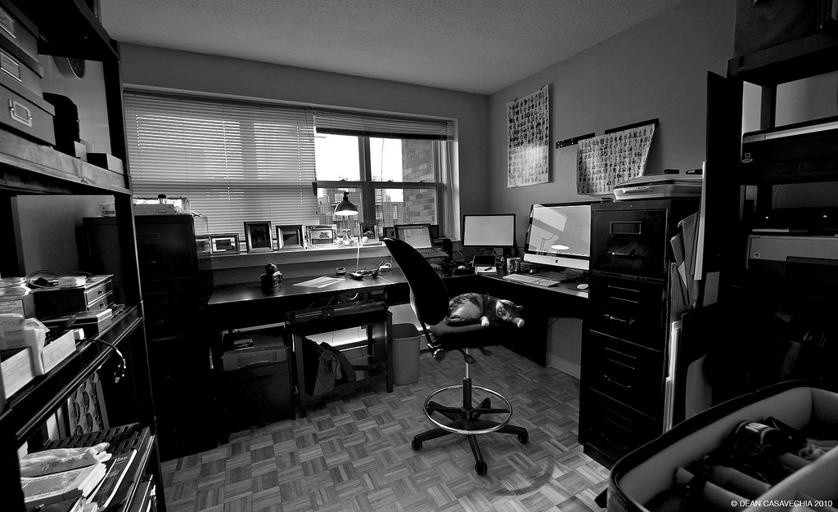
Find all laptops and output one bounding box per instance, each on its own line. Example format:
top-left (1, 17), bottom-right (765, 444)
top-left (394, 223), bottom-right (450, 258)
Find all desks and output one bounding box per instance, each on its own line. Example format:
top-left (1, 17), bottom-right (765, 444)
top-left (380, 261), bottom-right (588, 383)
top-left (206, 267), bottom-right (411, 432)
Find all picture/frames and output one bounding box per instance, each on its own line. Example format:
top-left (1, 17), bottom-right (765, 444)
top-left (306, 223), bottom-right (338, 247)
top-left (210, 232), bottom-right (241, 256)
top-left (243, 219), bottom-right (273, 253)
top-left (276, 224), bottom-right (304, 252)
top-left (431, 224), bottom-right (440, 240)
top-left (383, 226), bottom-right (396, 239)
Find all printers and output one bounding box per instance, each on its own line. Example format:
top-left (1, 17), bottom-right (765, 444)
top-left (612, 174), bottom-right (702, 201)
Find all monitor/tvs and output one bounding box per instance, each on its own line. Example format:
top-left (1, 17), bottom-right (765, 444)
top-left (462, 213), bottom-right (516, 254)
top-left (523, 200), bottom-right (601, 281)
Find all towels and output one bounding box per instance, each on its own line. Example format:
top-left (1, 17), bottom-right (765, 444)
top-left (16, 441), bottom-right (116, 479)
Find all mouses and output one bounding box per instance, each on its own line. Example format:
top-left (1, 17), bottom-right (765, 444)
top-left (577, 284), bottom-right (588, 289)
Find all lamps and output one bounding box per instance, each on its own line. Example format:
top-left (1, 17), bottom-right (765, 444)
top-left (332, 192), bottom-right (364, 281)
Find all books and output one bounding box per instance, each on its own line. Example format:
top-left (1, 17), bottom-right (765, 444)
top-left (23, 493), bottom-right (114, 512)
top-left (109, 451), bottom-right (162, 510)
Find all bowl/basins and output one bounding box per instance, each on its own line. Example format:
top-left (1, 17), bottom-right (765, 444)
top-left (337, 266), bottom-right (346, 274)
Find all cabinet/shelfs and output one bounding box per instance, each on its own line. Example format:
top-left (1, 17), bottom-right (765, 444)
top-left (701, 1), bottom-right (838, 426)
top-left (1, 0), bottom-right (168, 512)
top-left (576, 195), bottom-right (704, 476)
top-left (74, 210), bottom-right (227, 464)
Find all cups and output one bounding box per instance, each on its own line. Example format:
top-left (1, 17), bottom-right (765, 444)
top-left (507, 257), bottom-right (521, 274)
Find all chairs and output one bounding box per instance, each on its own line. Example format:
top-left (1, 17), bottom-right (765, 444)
top-left (382, 239), bottom-right (529, 478)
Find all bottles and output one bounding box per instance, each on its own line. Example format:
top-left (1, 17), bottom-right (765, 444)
top-left (158, 194), bottom-right (166, 204)
top-left (0, 277), bottom-right (38, 351)
top-left (342, 228), bottom-right (355, 246)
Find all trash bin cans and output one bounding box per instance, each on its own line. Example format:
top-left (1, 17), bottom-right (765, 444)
top-left (391, 323), bottom-right (422, 385)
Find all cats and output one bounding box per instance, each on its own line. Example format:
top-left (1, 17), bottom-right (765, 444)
top-left (443, 292), bottom-right (525, 328)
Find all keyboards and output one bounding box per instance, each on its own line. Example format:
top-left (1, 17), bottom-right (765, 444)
top-left (502, 274), bottom-right (560, 287)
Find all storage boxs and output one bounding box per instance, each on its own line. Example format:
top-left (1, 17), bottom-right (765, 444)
top-left (1, 2), bottom-right (45, 63)
top-left (1, 67), bottom-right (59, 150)
top-left (1, 30), bottom-right (47, 100)
top-left (305, 326), bottom-right (375, 383)
top-left (219, 336), bottom-right (295, 430)
